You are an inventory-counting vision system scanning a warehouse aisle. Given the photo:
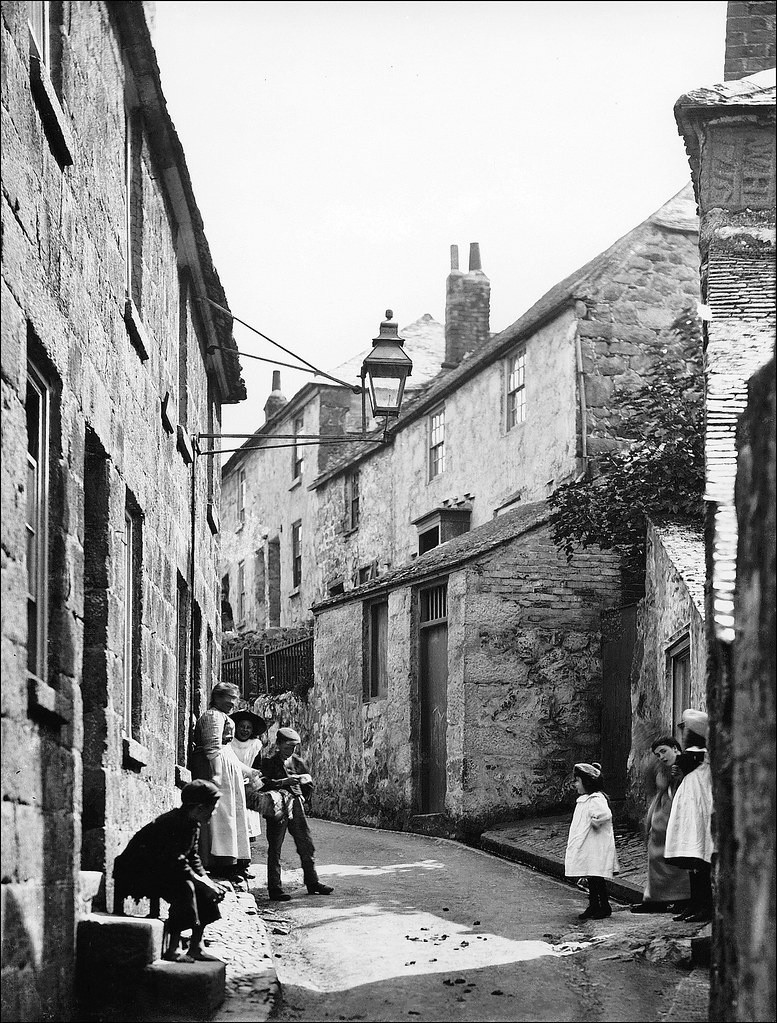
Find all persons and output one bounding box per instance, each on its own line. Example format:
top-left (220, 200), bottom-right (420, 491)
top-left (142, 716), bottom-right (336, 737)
top-left (111, 778), bottom-right (230, 964)
top-left (563, 762), bottom-right (618, 923)
top-left (252, 726), bottom-right (335, 902)
top-left (631, 708), bottom-right (718, 922)
top-left (193, 682), bottom-right (275, 886)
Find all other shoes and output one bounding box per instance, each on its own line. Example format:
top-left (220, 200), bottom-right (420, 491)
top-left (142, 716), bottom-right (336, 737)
top-left (579, 907), bottom-right (600, 919)
top-left (685, 914), bottom-right (713, 922)
top-left (592, 907), bottom-right (611, 918)
top-left (673, 911), bottom-right (694, 922)
top-left (269, 893), bottom-right (290, 901)
top-left (307, 881), bottom-right (333, 895)
top-left (239, 869), bottom-right (255, 879)
top-left (669, 904), bottom-right (688, 913)
top-left (224, 875), bottom-right (243, 883)
top-left (186, 951), bottom-right (216, 961)
top-left (630, 903), bottom-right (666, 913)
top-left (164, 950), bottom-right (193, 962)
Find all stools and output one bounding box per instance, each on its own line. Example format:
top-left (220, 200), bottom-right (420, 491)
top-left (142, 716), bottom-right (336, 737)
top-left (113, 880), bottom-right (163, 919)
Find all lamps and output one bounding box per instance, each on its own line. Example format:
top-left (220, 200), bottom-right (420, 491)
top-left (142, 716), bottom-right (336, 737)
top-left (191, 309), bottom-right (413, 458)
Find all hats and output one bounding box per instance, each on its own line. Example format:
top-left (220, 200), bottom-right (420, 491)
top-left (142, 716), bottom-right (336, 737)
top-left (181, 779), bottom-right (223, 805)
top-left (573, 763), bottom-right (602, 779)
top-left (277, 728), bottom-right (300, 745)
top-left (229, 710), bottom-right (266, 737)
top-left (677, 709), bottom-right (708, 738)
